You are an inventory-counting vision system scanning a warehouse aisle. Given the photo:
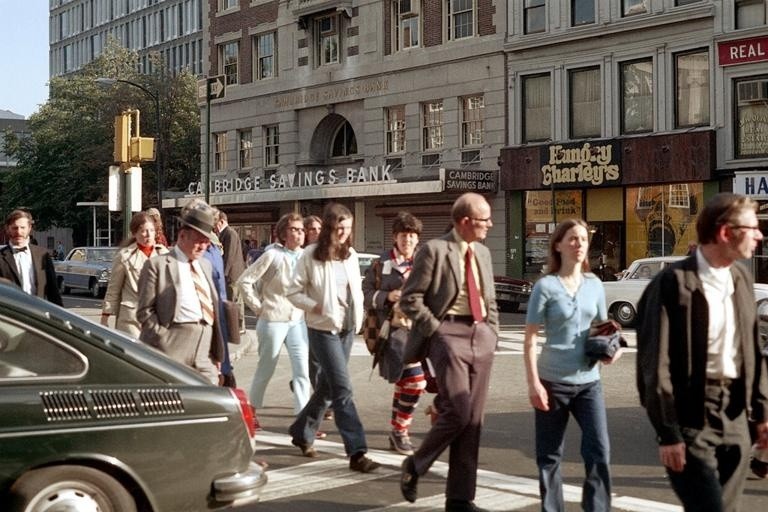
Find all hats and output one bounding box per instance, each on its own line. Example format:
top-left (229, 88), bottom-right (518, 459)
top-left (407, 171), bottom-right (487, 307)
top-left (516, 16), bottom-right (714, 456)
top-left (176, 209), bottom-right (222, 246)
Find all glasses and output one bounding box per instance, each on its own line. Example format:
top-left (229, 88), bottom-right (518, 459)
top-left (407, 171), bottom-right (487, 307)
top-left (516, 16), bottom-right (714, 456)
top-left (284, 228), bottom-right (304, 232)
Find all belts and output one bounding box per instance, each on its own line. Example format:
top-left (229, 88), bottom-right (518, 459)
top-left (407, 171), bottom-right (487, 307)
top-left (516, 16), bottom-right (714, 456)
top-left (442, 315), bottom-right (473, 322)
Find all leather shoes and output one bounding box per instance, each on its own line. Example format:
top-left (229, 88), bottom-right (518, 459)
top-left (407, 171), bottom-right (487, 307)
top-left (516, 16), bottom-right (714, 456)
top-left (402, 456), bottom-right (418, 502)
top-left (445, 501), bottom-right (490, 511)
top-left (350, 455), bottom-right (381, 471)
top-left (292, 438), bottom-right (319, 458)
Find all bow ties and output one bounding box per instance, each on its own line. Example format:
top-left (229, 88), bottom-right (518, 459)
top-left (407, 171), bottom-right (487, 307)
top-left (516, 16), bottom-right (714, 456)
top-left (15, 247), bottom-right (27, 252)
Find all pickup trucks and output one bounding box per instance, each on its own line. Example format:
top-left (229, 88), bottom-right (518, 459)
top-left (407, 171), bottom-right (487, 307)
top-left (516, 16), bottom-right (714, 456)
top-left (53, 245), bottom-right (122, 300)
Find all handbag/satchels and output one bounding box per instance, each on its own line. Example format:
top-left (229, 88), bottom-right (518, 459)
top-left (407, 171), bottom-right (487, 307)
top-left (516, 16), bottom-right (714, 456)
top-left (222, 298), bottom-right (240, 344)
top-left (358, 308), bottom-right (380, 352)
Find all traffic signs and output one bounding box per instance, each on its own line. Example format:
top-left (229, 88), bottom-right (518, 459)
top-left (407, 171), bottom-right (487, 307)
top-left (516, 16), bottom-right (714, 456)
top-left (197, 75), bottom-right (224, 101)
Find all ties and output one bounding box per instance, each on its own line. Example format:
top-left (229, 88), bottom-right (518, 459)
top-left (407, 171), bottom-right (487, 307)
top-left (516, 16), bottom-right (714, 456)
top-left (466, 246), bottom-right (483, 320)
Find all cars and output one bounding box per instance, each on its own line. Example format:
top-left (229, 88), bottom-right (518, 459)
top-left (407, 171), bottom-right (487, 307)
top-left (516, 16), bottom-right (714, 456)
top-left (589, 227), bottom-right (614, 263)
top-left (0, 279), bottom-right (268, 512)
top-left (352, 249), bottom-right (381, 284)
top-left (246, 247), bottom-right (268, 268)
top-left (1, 242), bottom-right (9, 252)
top-left (602, 255), bottom-right (768, 327)
top-left (493, 272), bottom-right (535, 313)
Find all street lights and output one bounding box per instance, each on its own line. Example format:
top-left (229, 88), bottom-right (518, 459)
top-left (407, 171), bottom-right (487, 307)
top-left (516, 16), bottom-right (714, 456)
top-left (97, 74), bottom-right (165, 232)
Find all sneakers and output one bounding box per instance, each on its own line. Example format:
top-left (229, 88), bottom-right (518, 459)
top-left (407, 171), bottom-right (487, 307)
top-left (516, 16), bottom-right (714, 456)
top-left (389, 430), bottom-right (414, 455)
top-left (324, 411), bottom-right (333, 419)
top-left (252, 416), bottom-right (262, 430)
top-left (315, 431), bottom-right (326, 438)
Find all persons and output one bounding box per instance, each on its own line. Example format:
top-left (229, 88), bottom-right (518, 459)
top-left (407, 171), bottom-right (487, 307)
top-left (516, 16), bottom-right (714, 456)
top-left (363, 211), bottom-right (428, 455)
top-left (634, 192), bottom-right (768, 512)
top-left (0, 209), bottom-right (65, 307)
top-left (524, 219), bottom-right (623, 511)
top-left (400, 192), bottom-right (501, 511)
